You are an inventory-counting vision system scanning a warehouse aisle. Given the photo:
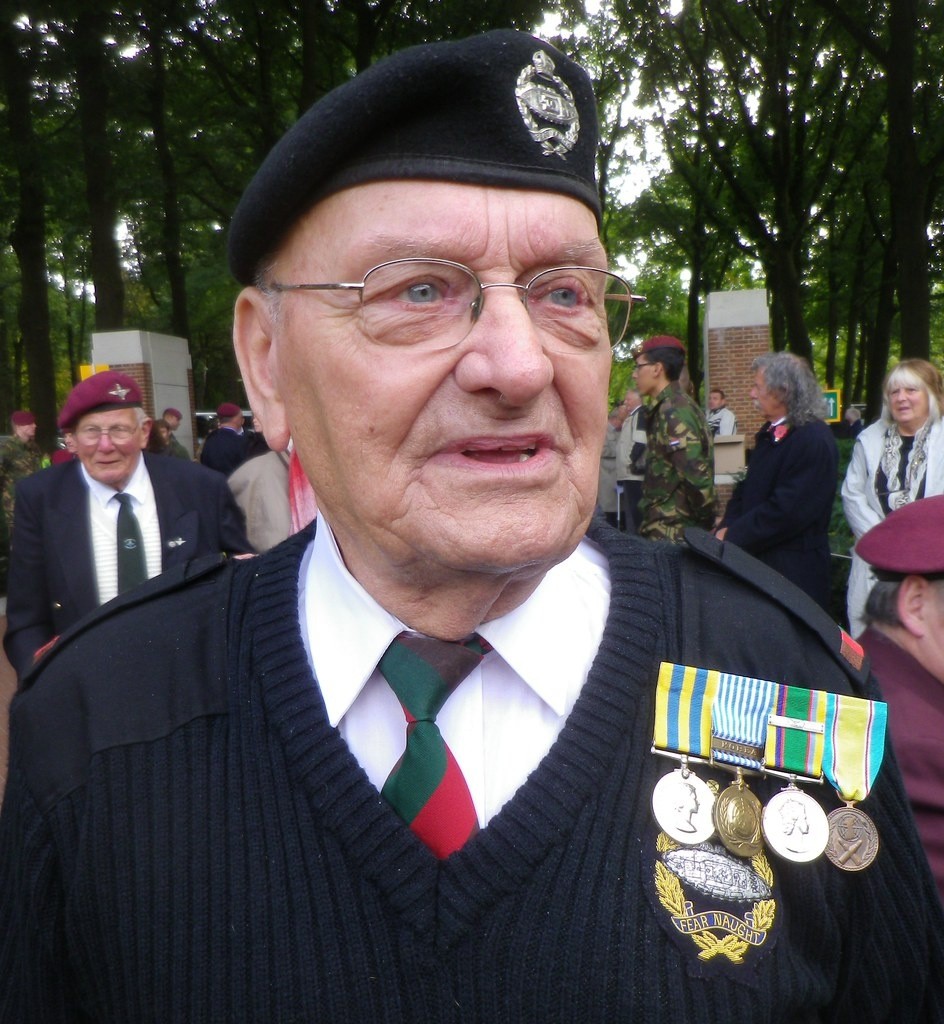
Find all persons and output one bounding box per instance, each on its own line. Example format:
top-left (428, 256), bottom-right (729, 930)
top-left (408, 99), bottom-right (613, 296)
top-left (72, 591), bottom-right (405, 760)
top-left (197, 402), bottom-right (271, 478)
top-left (844, 408), bottom-right (863, 438)
top-left (596, 406), bottom-right (628, 532)
top-left (53, 427), bottom-right (79, 463)
top-left (0, 412), bottom-right (45, 598)
top-left (630, 335), bottom-right (718, 545)
top-left (4, 370), bottom-right (258, 683)
top-left (145, 408), bottom-right (189, 458)
top-left (0, 27), bottom-right (944, 1024)
top-left (706, 389), bottom-right (737, 436)
top-left (615, 389), bottom-right (649, 537)
top-left (227, 434), bottom-right (319, 552)
top-left (853, 493), bottom-right (944, 901)
top-left (841, 359), bottom-right (944, 640)
top-left (714, 352), bottom-right (838, 613)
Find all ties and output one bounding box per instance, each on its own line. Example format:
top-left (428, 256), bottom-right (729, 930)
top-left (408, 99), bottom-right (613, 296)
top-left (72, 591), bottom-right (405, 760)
top-left (114, 492), bottom-right (148, 599)
top-left (378, 634), bottom-right (495, 861)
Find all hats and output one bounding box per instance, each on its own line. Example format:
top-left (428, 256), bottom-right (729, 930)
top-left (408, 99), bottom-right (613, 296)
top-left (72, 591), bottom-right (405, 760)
top-left (59, 372), bottom-right (143, 437)
top-left (216, 404), bottom-right (240, 417)
top-left (166, 407), bottom-right (181, 420)
top-left (12, 411), bottom-right (35, 427)
top-left (225, 32), bottom-right (601, 286)
top-left (629, 333), bottom-right (686, 358)
top-left (854, 491), bottom-right (944, 579)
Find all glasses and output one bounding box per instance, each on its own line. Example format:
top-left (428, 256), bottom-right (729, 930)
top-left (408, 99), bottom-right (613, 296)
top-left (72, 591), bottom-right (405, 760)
top-left (263, 258), bottom-right (650, 358)
top-left (67, 424), bottom-right (141, 448)
top-left (634, 358), bottom-right (659, 371)
